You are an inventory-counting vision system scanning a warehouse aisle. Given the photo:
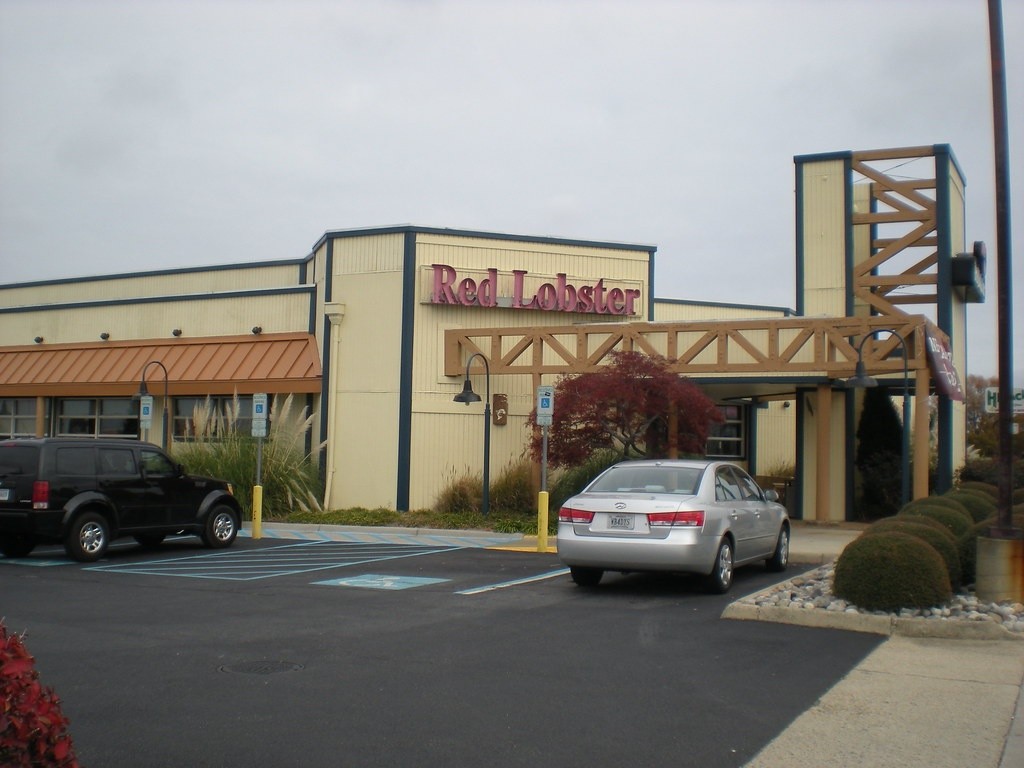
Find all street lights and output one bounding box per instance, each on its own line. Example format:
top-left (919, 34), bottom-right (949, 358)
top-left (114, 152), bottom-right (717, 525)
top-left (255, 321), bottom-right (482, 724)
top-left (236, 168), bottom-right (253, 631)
top-left (132, 361), bottom-right (169, 453)
top-left (844, 330), bottom-right (912, 506)
top-left (453, 353), bottom-right (491, 515)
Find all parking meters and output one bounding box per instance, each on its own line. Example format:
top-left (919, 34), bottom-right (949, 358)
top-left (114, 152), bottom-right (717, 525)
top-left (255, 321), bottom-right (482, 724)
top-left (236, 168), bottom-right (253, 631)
top-left (537, 385), bottom-right (554, 491)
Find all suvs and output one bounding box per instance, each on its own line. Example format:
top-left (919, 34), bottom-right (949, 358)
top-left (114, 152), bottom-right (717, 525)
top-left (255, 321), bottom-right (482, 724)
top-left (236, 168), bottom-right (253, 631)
top-left (0, 436), bottom-right (244, 564)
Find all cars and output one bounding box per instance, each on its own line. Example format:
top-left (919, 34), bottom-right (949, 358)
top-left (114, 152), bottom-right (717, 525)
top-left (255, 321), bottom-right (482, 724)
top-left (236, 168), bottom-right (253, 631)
top-left (556, 459), bottom-right (792, 595)
